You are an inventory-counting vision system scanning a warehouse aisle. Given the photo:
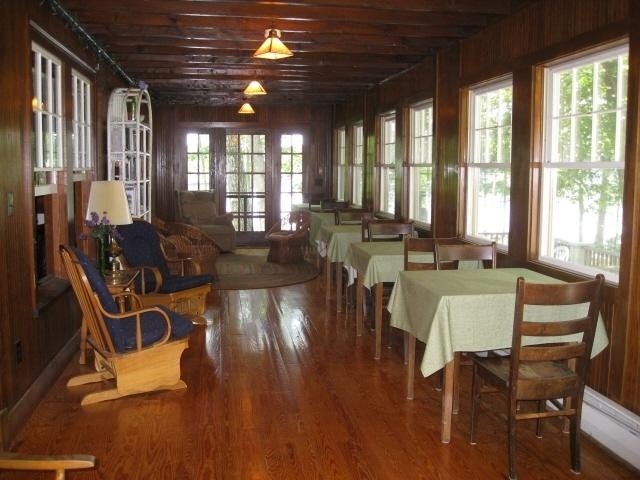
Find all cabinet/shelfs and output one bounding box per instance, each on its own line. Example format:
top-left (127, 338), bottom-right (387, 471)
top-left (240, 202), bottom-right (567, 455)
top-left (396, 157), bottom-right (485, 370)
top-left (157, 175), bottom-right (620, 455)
top-left (107, 88), bottom-right (153, 224)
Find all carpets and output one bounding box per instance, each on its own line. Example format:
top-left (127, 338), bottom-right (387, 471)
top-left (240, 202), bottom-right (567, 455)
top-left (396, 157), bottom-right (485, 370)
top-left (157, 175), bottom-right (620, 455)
top-left (211, 246), bottom-right (322, 290)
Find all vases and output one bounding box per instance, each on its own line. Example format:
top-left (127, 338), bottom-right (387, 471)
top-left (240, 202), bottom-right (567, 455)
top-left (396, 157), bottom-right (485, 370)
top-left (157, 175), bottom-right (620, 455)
top-left (96, 237), bottom-right (108, 279)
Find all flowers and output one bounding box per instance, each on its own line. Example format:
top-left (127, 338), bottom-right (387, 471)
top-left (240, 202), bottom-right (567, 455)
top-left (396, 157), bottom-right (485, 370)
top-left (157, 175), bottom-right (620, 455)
top-left (79, 211), bottom-right (127, 241)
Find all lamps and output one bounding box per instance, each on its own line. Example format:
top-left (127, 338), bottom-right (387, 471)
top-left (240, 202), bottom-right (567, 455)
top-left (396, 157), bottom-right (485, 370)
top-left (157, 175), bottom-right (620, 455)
top-left (244, 70), bottom-right (267, 96)
top-left (238, 99), bottom-right (255, 114)
top-left (84, 180), bottom-right (133, 271)
top-left (253, 18), bottom-right (294, 60)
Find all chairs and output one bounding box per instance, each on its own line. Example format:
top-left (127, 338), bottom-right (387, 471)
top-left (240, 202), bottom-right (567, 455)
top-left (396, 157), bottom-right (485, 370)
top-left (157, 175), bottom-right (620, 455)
top-left (60, 245), bottom-right (195, 405)
top-left (113, 218), bottom-right (214, 326)
top-left (0, 451), bottom-right (95, 479)
top-left (152, 216), bottom-right (223, 281)
top-left (174, 190), bottom-right (237, 252)
top-left (264, 209), bottom-right (311, 264)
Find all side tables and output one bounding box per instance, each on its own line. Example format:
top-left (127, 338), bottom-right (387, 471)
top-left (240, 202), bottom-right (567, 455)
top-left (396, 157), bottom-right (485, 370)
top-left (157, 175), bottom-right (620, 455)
top-left (78, 269), bottom-right (140, 365)
